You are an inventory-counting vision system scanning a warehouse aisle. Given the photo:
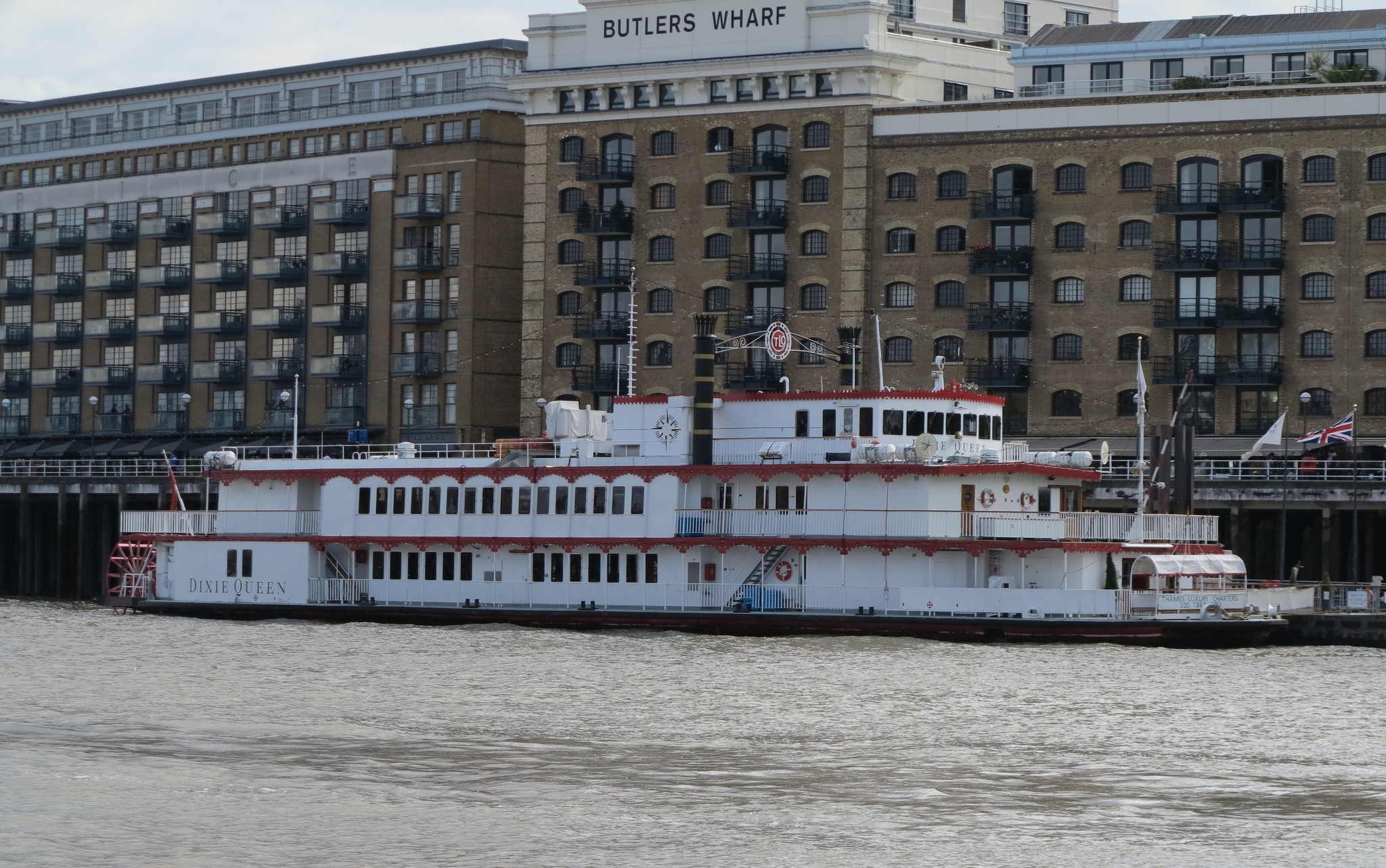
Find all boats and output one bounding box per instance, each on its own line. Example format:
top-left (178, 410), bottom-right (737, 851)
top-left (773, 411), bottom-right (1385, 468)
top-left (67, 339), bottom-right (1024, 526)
top-left (102, 264), bottom-right (1290, 653)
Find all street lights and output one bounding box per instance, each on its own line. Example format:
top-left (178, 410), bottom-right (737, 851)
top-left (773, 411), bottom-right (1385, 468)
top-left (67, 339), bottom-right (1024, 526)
top-left (89, 396), bottom-right (99, 459)
top-left (1, 399), bottom-right (11, 476)
top-left (279, 391), bottom-right (291, 458)
top-left (403, 399), bottom-right (415, 442)
top-left (1133, 393), bottom-right (1140, 462)
top-left (182, 393), bottom-right (192, 464)
top-left (536, 398), bottom-right (547, 438)
top-left (1298, 392), bottom-right (1312, 453)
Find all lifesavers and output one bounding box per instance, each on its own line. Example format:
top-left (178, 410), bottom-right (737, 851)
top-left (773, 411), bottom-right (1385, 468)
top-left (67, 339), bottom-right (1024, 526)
top-left (981, 488), bottom-right (995, 507)
top-left (776, 561), bottom-right (791, 581)
top-left (1021, 490), bottom-right (1034, 509)
top-left (870, 441), bottom-right (881, 445)
top-left (1362, 588), bottom-right (1374, 604)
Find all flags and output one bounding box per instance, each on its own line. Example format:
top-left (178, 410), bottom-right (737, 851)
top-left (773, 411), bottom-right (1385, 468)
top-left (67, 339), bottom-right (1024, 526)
top-left (1136, 347), bottom-right (1147, 403)
top-left (1292, 410), bottom-right (1353, 443)
top-left (168, 470), bottom-right (178, 512)
top-left (1240, 411), bottom-right (1287, 461)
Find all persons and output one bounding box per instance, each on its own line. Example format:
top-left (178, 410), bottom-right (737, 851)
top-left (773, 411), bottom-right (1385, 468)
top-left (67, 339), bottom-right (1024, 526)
top-left (1320, 572), bottom-right (1331, 612)
top-left (1296, 563), bottom-right (1303, 580)
top-left (111, 403), bottom-right (118, 429)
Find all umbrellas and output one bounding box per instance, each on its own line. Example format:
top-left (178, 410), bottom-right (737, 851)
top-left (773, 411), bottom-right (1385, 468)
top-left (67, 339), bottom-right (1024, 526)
top-left (0, 435), bottom-right (328, 458)
